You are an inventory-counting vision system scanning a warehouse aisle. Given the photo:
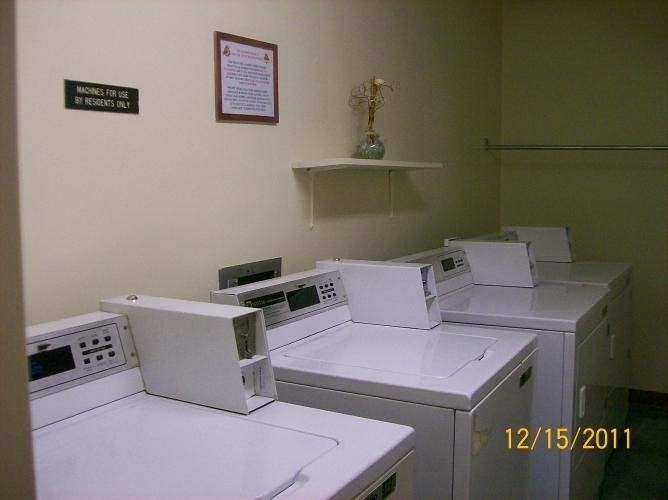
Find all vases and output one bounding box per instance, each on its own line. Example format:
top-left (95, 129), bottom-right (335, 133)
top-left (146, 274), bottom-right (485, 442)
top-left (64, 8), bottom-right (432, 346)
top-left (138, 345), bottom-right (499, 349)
top-left (357, 132), bottom-right (385, 159)
